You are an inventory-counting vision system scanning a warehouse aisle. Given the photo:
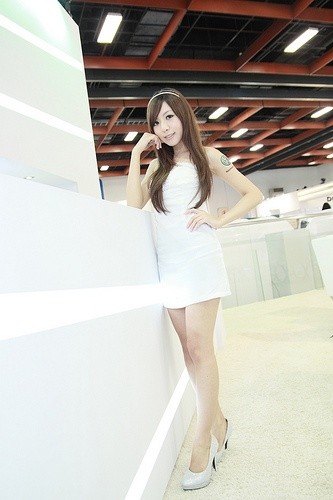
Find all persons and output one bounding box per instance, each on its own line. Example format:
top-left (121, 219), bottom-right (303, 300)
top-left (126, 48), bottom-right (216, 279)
top-left (126, 89), bottom-right (266, 491)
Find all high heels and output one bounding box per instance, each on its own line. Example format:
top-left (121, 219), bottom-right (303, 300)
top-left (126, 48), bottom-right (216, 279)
top-left (182, 434), bottom-right (219, 490)
top-left (216, 419), bottom-right (230, 462)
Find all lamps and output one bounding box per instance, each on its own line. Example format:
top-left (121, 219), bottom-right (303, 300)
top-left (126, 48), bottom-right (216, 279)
top-left (311, 106), bottom-right (333, 118)
top-left (249, 144), bottom-right (264, 151)
top-left (322, 142), bottom-right (333, 149)
top-left (100, 164), bottom-right (110, 172)
top-left (231, 128), bottom-right (248, 138)
top-left (125, 131), bottom-right (139, 142)
top-left (96, 12), bottom-right (123, 44)
top-left (282, 26), bottom-right (320, 54)
top-left (209, 106), bottom-right (228, 120)
